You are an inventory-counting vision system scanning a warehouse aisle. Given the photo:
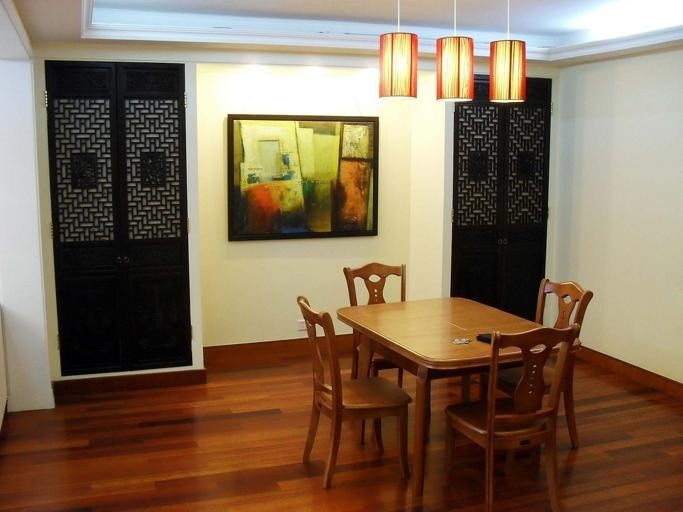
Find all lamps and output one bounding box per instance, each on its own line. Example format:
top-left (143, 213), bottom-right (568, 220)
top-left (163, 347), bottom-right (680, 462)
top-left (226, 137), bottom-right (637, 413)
top-left (374, 1), bottom-right (422, 100)
top-left (487, 0), bottom-right (526, 105)
top-left (432, 1), bottom-right (476, 103)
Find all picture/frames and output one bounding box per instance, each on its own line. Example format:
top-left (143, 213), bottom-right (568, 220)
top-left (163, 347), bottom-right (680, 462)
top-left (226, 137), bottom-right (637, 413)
top-left (225, 112), bottom-right (380, 243)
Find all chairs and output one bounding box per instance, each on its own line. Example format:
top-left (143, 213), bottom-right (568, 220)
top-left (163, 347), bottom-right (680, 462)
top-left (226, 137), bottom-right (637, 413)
top-left (295, 293), bottom-right (411, 492)
top-left (342, 262), bottom-right (408, 392)
top-left (476, 277), bottom-right (594, 452)
top-left (442, 322), bottom-right (582, 512)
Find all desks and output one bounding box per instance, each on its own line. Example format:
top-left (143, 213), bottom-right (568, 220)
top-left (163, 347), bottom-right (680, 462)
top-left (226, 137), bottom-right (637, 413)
top-left (333, 295), bottom-right (582, 497)
